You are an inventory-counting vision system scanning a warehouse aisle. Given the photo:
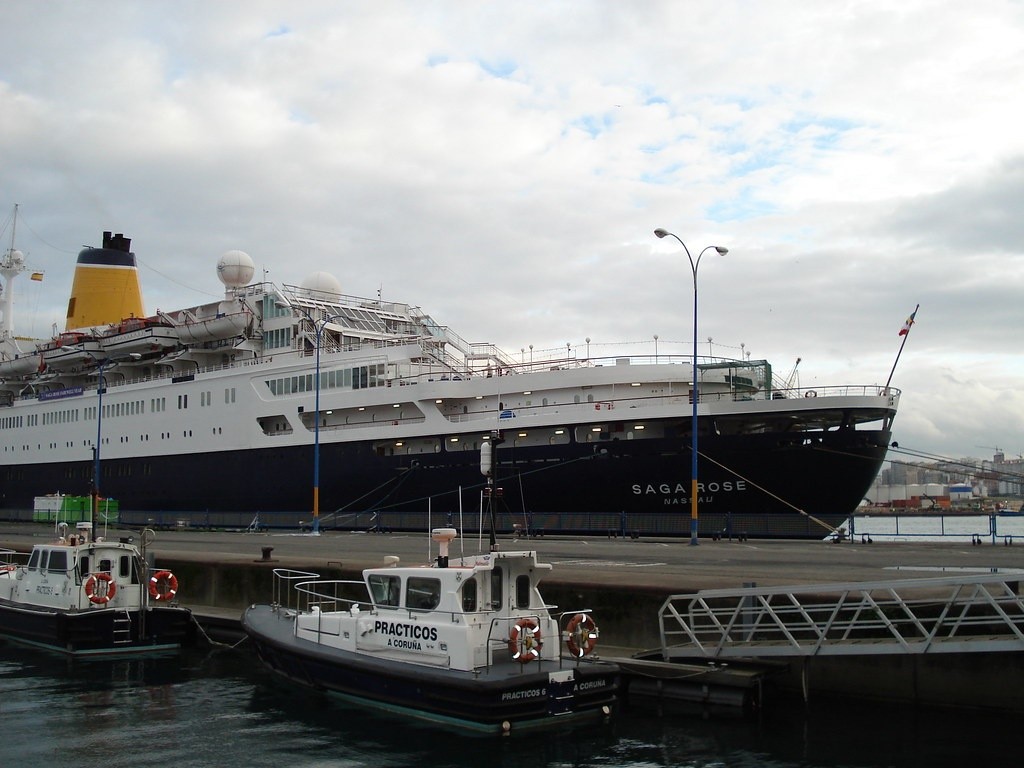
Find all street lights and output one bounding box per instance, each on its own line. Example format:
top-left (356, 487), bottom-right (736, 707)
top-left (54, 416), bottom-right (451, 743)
top-left (654, 226), bottom-right (729, 546)
top-left (274, 299), bottom-right (361, 534)
top-left (62, 344), bottom-right (142, 542)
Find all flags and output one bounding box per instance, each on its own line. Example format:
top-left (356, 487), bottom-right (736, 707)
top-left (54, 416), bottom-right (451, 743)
top-left (31, 273), bottom-right (43, 281)
top-left (900, 312), bottom-right (914, 335)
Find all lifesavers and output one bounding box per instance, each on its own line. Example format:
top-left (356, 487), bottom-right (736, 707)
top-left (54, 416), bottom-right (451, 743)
top-left (507, 619), bottom-right (544, 664)
top-left (85, 573), bottom-right (117, 605)
top-left (147, 571), bottom-right (178, 603)
top-left (565, 612), bottom-right (598, 657)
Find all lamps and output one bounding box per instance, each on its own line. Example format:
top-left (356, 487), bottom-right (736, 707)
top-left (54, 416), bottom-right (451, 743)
top-left (472, 351), bottom-right (474, 355)
top-left (741, 343), bottom-right (745, 347)
top-left (746, 351), bottom-right (751, 355)
top-left (120, 535), bottom-right (134, 544)
top-left (465, 353), bottom-right (468, 357)
top-left (521, 348), bottom-right (524, 352)
top-left (653, 334), bottom-right (659, 340)
top-left (567, 343), bottom-right (570, 346)
top-left (708, 337), bottom-right (712, 341)
top-left (529, 344), bottom-right (533, 349)
top-left (586, 337), bottom-right (591, 342)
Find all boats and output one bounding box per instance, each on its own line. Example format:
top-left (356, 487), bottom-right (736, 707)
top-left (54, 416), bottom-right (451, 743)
top-left (240, 486), bottom-right (620, 746)
top-left (0, 519), bottom-right (194, 666)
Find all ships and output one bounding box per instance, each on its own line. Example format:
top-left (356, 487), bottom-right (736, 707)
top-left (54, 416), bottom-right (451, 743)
top-left (0, 204), bottom-right (923, 538)
